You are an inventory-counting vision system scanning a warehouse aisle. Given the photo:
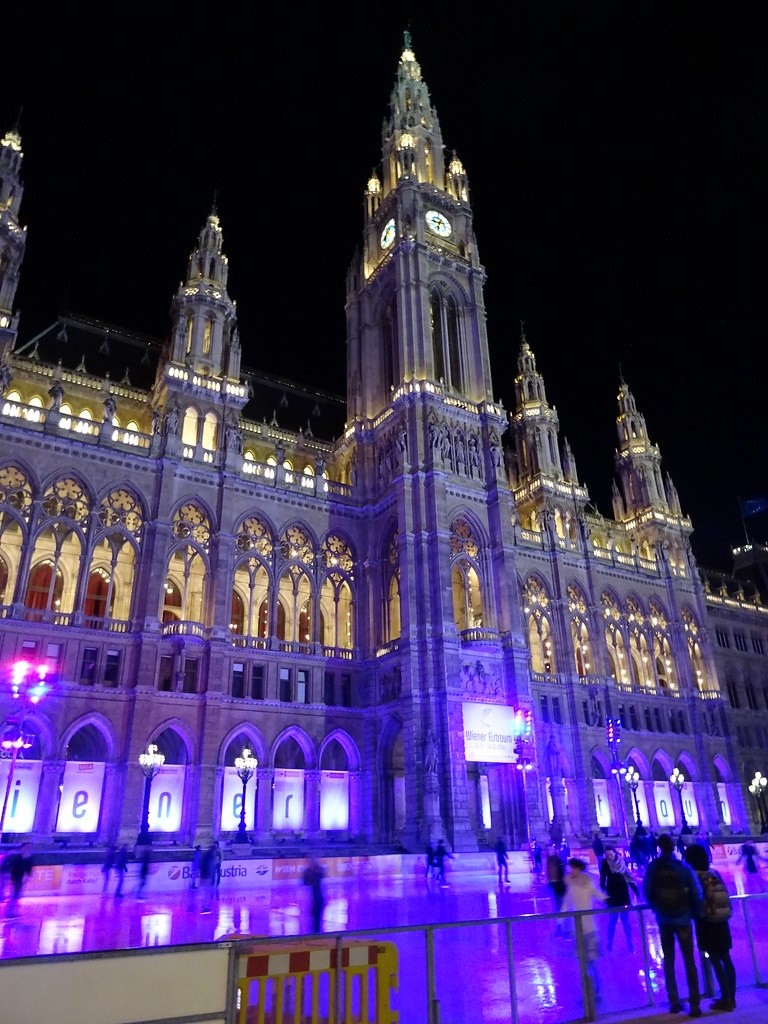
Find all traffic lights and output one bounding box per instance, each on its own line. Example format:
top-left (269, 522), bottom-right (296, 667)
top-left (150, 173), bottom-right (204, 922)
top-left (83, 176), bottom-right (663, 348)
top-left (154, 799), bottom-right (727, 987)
top-left (614, 717), bottom-right (622, 745)
top-left (513, 706), bottom-right (524, 739)
top-left (524, 709), bottom-right (532, 737)
top-left (605, 715), bottom-right (614, 747)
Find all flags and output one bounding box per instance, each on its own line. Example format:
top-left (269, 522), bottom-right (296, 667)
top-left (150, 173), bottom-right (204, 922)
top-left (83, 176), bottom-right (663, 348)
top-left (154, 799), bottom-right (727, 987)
top-left (737, 494), bottom-right (768, 521)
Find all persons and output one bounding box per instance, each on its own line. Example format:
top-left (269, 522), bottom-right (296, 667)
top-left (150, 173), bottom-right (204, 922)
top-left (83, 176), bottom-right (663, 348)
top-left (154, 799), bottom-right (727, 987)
top-left (302, 854), bottom-right (329, 935)
top-left (379, 425), bottom-right (407, 464)
top-left (600, 846), bottom-right (638, 951)
top-left (476, 659), bottom-right (485, 683)
top-left (685, 844), bottom-right (736, 1012)
top-left (0, 364), bottom-right (178, 435)
top-left (547, 847), bottom-right (568, 912)
top-left (644, 833), bottom-right (704, 1018)
top-left (494, 836), bottom-right (511, 885)
top-left (0, 841), bottom-right (34, 918)
top-left (191, 841), bottom-right (222, 889)
top-left (225, 426), bottom-right (357, 486)
top-left (101, 844), bottom-right (154, 900)
top-left (533, 831), bottom-right (768, 875)
top-left (544, 735), bottom-right (563, 776)
top-left (431, 422), bottom-right (502, 469)
top-left (419, 727), bottom-right (439, 775)
top-left (511, 501), bottom-right (696, 568)
top-left (556, 858), bottom-right (610, 962)
top-left (425, 839), bottom-right (455, 888)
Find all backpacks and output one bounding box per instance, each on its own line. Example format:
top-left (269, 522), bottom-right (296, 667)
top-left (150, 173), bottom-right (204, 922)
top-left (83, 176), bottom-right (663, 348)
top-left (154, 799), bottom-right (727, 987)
top-left (698, 871), bottom-right (732, 922)
top-left (649, 858), bottom-right (690, 913)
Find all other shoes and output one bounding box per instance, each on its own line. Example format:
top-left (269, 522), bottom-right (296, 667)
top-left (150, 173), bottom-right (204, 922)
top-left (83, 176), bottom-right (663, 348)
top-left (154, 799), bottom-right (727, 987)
top-left (671, 1004), bottom-right (683, 1012)
top-left (716, 999), bottom-right (736, 1007)
top-left (711, 1004), bottom-right (733, 1011)
top-left (691, 1008), bottom-right (702, 1017)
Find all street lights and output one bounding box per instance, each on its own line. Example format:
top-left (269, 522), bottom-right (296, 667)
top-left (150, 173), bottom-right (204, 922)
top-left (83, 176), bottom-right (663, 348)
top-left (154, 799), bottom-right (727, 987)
top-left (669, 767), bottom-right (694, 834)
top-left (747, 770), bottom-right (768, 835)
top-left (515, 763), bottom-right (534, 843)
top-left (233, 740), bottom-right (259, 845)
top-left (610, 767), bottom-right (632, 849)
top-left (138, 738), bottom-right (166, 847)
top-left (625, 772), bottom-right (648, 835)
top-left (0, 729), bottom-right (35, 848)
